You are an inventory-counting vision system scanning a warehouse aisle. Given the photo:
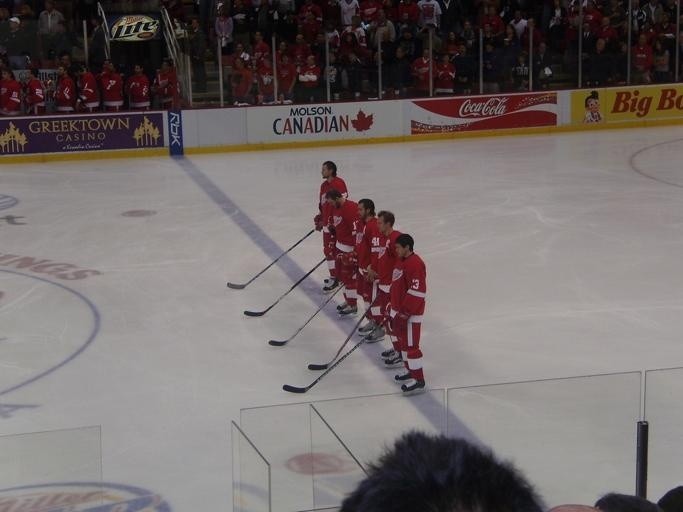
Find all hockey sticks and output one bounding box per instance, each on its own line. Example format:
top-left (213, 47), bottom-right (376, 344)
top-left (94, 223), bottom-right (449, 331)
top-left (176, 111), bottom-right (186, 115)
top-left (268, 271), bottom-right (357, 345)
top-left (227, 228), bottom-right (317, 288)
top-left (308, 293), bottom-right (381, 370)
top-left (244, 251), bottom-right (334, 316)
top-left (283, 316), bottom-right (387, 393)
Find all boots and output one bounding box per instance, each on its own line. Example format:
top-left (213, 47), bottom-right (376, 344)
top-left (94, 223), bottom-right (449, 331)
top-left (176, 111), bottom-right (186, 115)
top-left (323, 278), bottom-right (339, 290)
top-left (359, 322), bottom-right (385, 340)
top-left (337, 302), bottom-right (357, 314)
top-left (395, 372), bottom-right (425, 392)
top-left (381, 349), bottom-right (403, 364)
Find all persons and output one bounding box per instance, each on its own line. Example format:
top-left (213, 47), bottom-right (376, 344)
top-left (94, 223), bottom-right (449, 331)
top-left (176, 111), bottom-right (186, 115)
top-left (338, 428), bottom-right (682, 511)
top-left (2, 1), bottom-right (681, 118)
top-left (314, 161), bottom-right (427, 393)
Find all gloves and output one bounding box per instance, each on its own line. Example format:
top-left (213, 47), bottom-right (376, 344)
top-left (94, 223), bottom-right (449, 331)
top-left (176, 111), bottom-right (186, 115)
top-left (385, 304), bottom-right (410, 320)
top-left (358, 264), bottom-right (368, 280)
top-left (324, 247), bottom-right (337, 260)
top-left (314, 215), bottom-right (323, 231)
top-left (342, 254), bottom-right (355, 266)
